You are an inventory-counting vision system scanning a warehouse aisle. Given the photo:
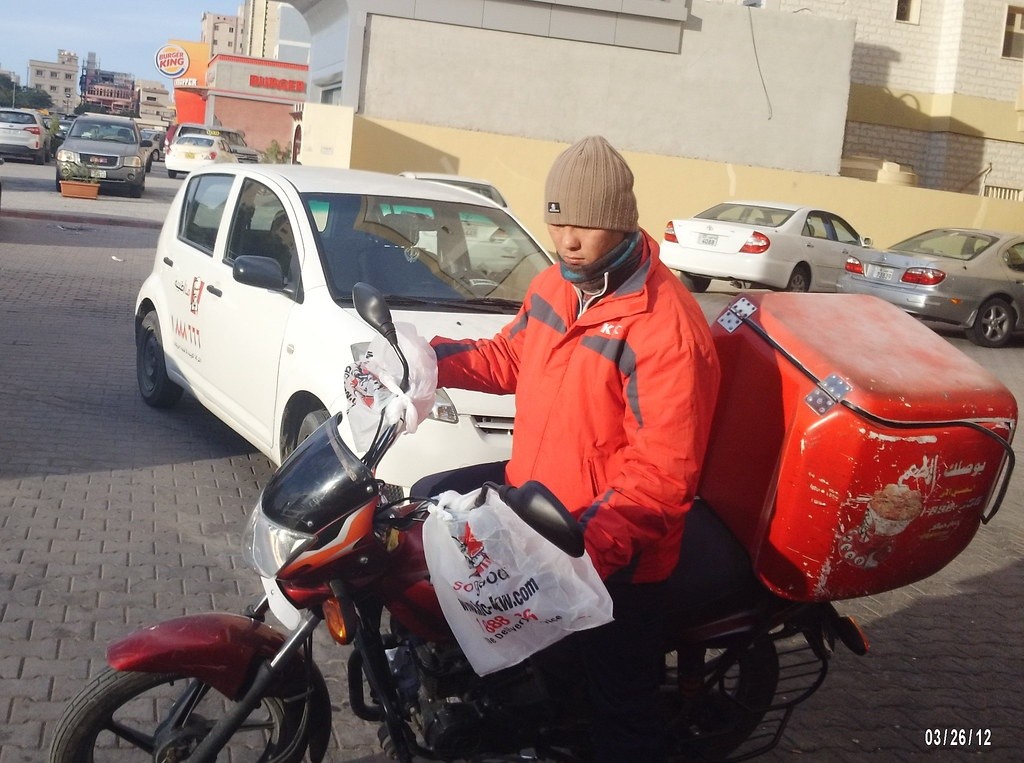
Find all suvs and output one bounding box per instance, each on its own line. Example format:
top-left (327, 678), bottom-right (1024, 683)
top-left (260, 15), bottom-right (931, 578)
top-left (0, 107), bottom-right (52, 165)
top-left (140, 129), bottom-right (167, 162)
top-left (132, 162), bottom-right (556, 510)
top-left (54, 113), bottom-right (154, 198)
top-left (170, 122), bottom-right (259, 163)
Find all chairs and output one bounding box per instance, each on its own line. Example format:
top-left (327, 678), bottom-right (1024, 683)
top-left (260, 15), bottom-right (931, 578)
top-left (265, 209), bottom-right (299, 282)
top-left (358, 214), bottom-right (453, 292)
top-left (804, 224), bottom-right (815, 236)
top-left (755, 217), bottom-right (773, 225)
top-left (80, 126), bottom-right (99, 135)
top-left (117, 129), bottom-right (134, 142)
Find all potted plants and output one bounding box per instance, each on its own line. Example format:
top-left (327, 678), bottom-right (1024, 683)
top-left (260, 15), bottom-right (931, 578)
top-left (59, 155), bottom-right (101, 199)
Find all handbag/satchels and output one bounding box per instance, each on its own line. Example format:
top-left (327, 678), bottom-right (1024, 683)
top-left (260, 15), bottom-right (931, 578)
top-left (342, 317), bottom-right (439, 436)
top-left (421, 490), bottom-right (615, 677)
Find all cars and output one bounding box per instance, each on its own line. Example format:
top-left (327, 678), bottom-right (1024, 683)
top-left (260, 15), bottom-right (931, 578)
top-left (41, 111), bottom-right (100, 141)
top-left (164, 133), bottom-right (241, 178)
top-left (381, 171), bottom-right (522, 276)
top-left (835, 228), bottom-right (1024, 348)
top-left (659, 201), bottom-right (879, 294)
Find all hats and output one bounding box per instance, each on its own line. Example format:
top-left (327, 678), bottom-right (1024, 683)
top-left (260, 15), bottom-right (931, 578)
top-left (543, 136), bottom-right (640, 234)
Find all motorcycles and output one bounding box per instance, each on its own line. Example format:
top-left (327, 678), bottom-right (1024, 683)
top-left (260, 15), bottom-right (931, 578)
top-left (44, 283), bottom-right (1021, 763)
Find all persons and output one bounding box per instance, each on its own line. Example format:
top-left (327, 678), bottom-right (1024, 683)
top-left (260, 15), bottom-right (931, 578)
top-left (348, 135), bottom-right (720, 763)
